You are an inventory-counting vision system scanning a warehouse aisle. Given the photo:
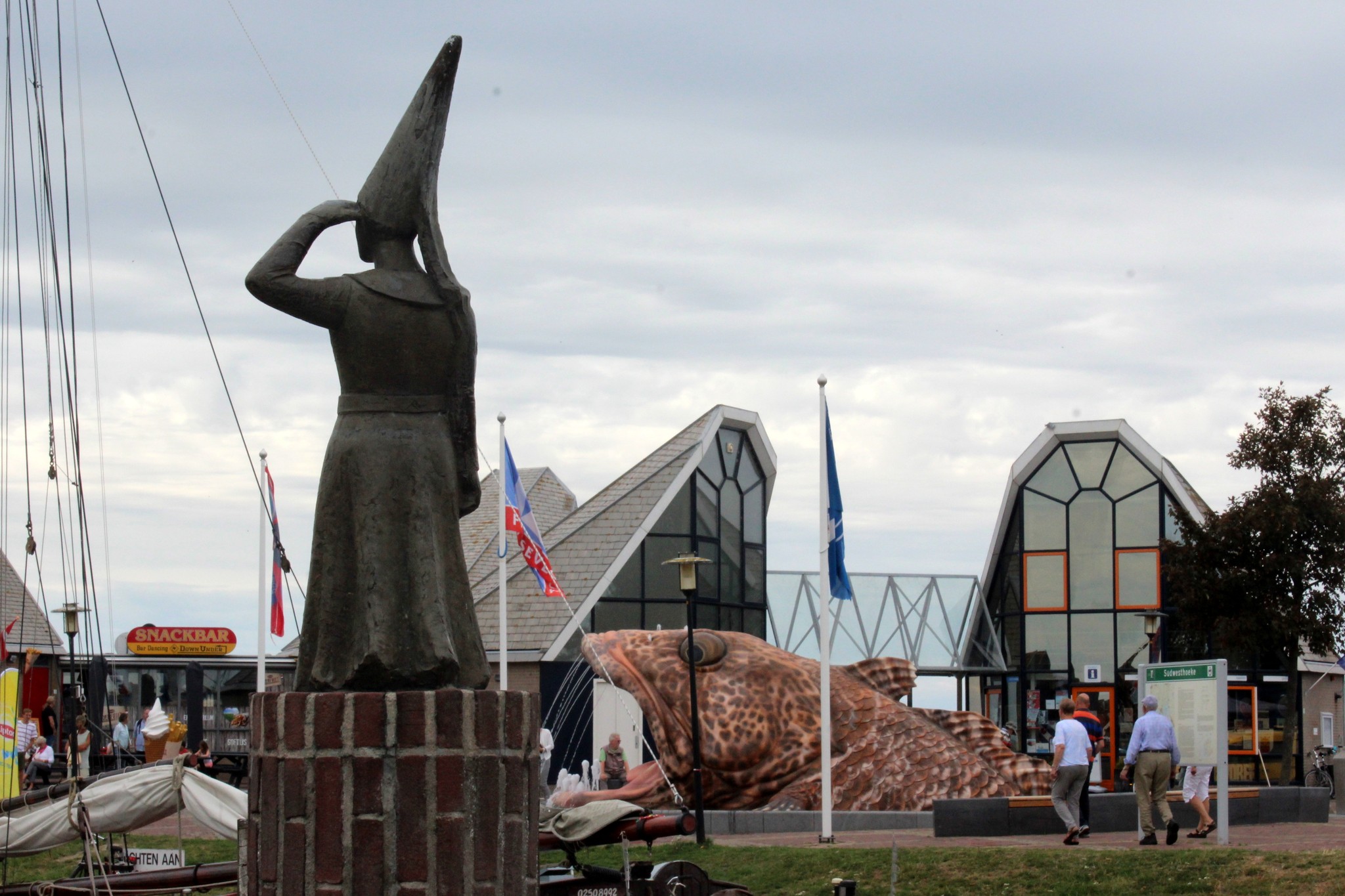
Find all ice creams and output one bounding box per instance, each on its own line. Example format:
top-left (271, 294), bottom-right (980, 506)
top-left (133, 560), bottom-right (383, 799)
top-left (141, 697), bottom-right (170, 764)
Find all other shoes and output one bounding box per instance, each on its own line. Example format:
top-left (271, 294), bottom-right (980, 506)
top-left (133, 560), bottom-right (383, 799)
top-left (1078, 825), bottom-right (1090, 837)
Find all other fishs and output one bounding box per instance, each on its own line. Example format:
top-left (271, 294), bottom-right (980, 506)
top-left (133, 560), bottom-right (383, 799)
top-left (554, 627), bottom-right (1058, 815)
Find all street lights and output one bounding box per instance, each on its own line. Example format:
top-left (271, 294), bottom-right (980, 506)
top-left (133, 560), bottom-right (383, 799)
top-left (51, 602), bottom-right (93, 777)
top-left (1134, 609), bottom-right (1169, 664)
top-left (663, 550), bottom-right (713, 850)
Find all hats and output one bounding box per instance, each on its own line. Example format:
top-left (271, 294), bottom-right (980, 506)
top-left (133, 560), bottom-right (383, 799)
top-left (1005, 722), bottom-right (1017, 736)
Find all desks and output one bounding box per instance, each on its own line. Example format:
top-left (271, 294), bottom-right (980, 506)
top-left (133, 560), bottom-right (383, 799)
top-left (209, 753), bottom-right (249, 788)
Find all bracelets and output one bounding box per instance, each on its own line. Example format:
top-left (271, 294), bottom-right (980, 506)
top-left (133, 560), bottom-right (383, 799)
top-left (542, 748), bottom-right (545, 754)
top-left (1122, 768), bottom-right (1128, 771)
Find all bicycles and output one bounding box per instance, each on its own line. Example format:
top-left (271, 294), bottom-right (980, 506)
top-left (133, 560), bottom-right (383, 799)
top-left (70, 829), bottom-right (138, 878)
top-left (1304, 744), bottom-right (1336, 800)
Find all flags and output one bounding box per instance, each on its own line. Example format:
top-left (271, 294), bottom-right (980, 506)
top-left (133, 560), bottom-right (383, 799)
top-left (505, 432), bottom-right (566, 597)
top-left (261, 464), bottom-right (284, 637)
top-left (1337, 655), bottom-right (1345, 670)
top-left (0, 618), bottom-right (17, 661)
top-left (820, 389), bottom-right (854, 601)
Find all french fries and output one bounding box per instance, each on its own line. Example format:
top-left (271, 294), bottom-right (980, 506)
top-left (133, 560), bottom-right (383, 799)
top-left (166, 713), bottom-right (188, 742)
top-left (26, 647), bottom-right (42, 655)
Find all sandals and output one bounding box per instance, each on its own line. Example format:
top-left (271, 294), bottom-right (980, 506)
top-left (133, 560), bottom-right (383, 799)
top-left (1063, 826), bottom-right (1079, 845)
top-left (1187, 820), bottom-right (1217, 838)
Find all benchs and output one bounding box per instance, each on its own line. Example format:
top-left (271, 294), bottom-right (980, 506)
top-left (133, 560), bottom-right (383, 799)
top-left (210, 763), bottom-right (247, 775)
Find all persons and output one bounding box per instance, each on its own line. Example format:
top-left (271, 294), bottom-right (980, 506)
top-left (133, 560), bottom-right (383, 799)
top-left (1000, 722), bottom-right (1018, 750)
top-left (1176, 765), bottom-right (1216, 838)
top-left (1119, 696), bottom-right (1181, 845)
top-left (16, 695), bottom-right (212, 793)
top-left (539, 717), bottom-right (554, 802)
top-left (245, 34), bottom-right (491, 692)
top-left (596, 733), bottom-right (630, 791)
top-left (1050, 698), bottom-right (1092, 845)
top-left (1072, 693), bottom-right (1104, 839)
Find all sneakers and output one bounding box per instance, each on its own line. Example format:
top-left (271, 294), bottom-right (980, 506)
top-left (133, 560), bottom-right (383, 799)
top-left (1166, 821), bottom-right (1179, 845)
top-left (1139, 833), bottom-right (1157, 845)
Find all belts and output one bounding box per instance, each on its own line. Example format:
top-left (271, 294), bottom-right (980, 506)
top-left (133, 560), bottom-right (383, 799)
top-left (1141, 749), bottom-right (1169, 753)
